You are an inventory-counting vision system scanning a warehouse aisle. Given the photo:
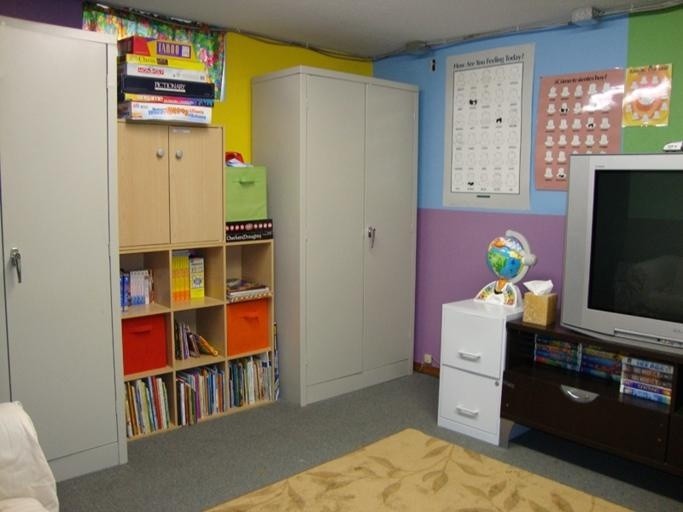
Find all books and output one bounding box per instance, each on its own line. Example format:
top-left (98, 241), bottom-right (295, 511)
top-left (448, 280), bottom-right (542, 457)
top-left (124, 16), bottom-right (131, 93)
top-left (224, 276), bottom-right (269, 298)
top-left (530, 332), bottom-right (673, 409)
top-left (118, 266), bottom-right (153, 313)
top-left (174, 318), bottom-right (218, 360)
top-left (123, 351), bottom-right (276, 441)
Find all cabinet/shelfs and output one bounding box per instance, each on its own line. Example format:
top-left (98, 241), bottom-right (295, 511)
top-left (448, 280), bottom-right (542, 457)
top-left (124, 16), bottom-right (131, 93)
top-left (504, 311), bottom-right (682, 490)
top-left (437, 297), bottom-right (529, 447)
top-left (250, 65), bottom-right (421, 406)
top-left (114, 115), bottom-right (279, 443)
top-left (0, 16), bottom-right (127, 483)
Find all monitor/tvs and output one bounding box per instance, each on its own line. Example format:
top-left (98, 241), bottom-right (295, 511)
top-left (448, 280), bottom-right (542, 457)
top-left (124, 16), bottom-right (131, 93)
top-left (560, 152), bottom-right (683, 355)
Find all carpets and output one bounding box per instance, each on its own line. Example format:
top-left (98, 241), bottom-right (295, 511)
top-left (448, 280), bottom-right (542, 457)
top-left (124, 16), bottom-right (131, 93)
top-left (206, 426), bottom-right (634, 510)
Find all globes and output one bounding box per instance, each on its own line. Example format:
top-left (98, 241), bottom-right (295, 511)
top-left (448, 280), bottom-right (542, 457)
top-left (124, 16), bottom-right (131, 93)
top-left (472, 229), bottom-right (537, 308)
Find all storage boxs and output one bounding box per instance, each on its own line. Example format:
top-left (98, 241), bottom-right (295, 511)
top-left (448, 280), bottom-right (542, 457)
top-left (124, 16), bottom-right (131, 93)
top-left (224, 161), bottom-right (272, 243)
top-left (115, 34), bottom-right (213, 126)
top-left (125, 311), bottom-right (166, 378)
top-left (224, 298), bottom-right (271, 356)
